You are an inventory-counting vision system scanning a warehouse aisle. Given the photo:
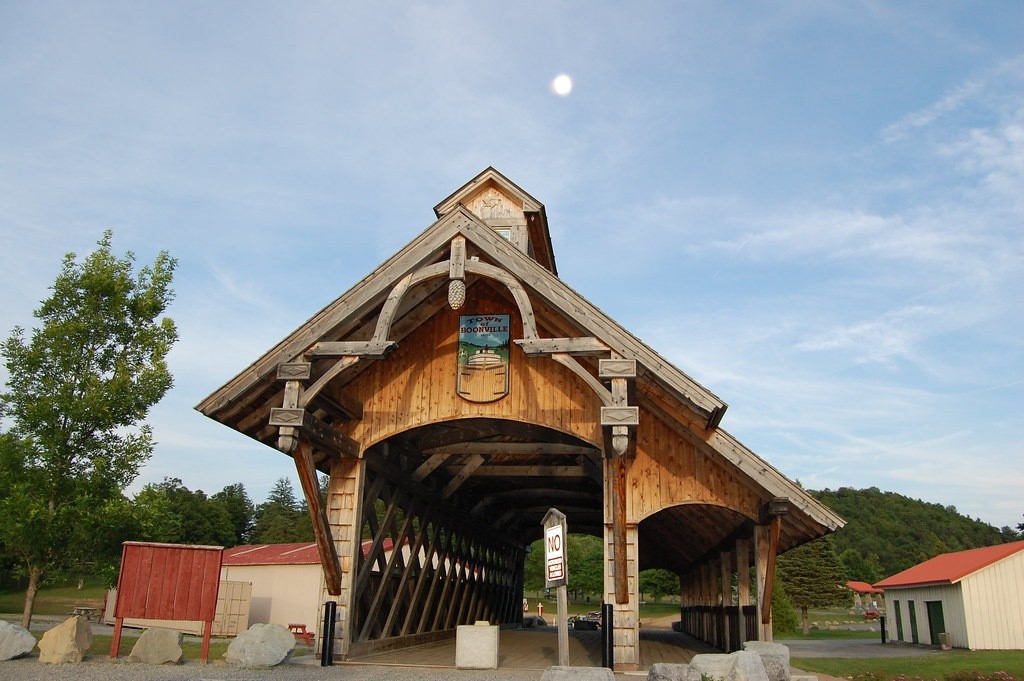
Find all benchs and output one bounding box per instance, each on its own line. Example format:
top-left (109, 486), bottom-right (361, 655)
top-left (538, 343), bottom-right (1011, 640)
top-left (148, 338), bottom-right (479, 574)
top-left (68, 607), bottom-right (97, 620)
top-left (288, 624), bottom-right (311, 647)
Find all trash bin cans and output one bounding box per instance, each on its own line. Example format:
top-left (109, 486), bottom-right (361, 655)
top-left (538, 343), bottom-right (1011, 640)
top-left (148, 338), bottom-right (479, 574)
top-left (455, 621), bottom-right (500, 669)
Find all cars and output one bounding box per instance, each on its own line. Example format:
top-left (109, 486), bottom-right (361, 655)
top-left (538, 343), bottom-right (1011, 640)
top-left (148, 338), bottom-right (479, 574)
top-left (568, 610), bottom-right (603, 626)
